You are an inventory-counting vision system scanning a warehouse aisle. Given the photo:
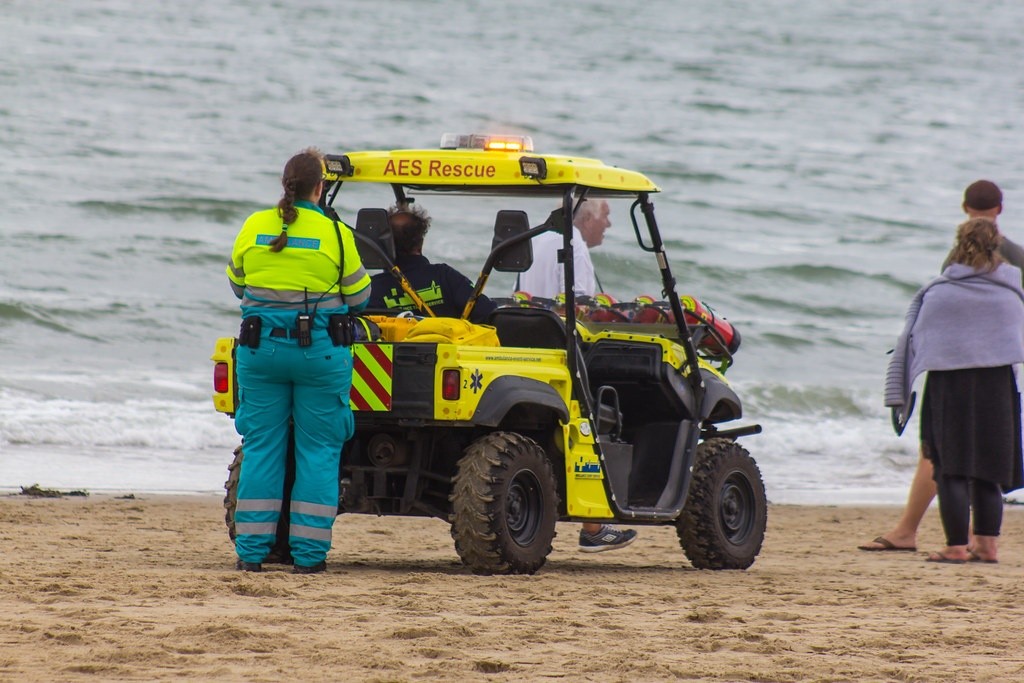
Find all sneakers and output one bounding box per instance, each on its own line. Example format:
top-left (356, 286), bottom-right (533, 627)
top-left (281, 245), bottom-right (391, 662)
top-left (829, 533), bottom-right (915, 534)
top-left (578, 525), bottom-right (638, 553)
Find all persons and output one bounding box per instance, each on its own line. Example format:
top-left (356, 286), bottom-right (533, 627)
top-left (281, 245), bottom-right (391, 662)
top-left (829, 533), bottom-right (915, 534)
top-left (858, 180), bottom-right (1024, 552)
top-left (883, 222), bottom-right (1024, 565)
top-left (514, 197), bottom-right (637, 553)
top-left (226, 154), bottom-right (372, 574)
top-left (368, 206), bottom-right (497, 324)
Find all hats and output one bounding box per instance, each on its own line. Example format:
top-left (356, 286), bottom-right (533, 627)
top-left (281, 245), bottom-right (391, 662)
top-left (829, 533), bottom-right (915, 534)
top-left (965, 180), bottom-right (1002, 210)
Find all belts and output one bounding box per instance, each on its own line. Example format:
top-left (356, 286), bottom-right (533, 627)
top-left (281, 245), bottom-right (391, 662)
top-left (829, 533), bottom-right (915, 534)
top-left (270, 327), bottom-right (298, 338)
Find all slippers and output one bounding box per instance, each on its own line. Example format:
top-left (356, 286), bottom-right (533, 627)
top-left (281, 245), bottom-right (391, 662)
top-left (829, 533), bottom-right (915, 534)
top-left (925, 551), bottom-right (966, 563)
top-left (857, 536), bottom-right (917, 551)
top-left (967, 549), bottom-right (999, 563)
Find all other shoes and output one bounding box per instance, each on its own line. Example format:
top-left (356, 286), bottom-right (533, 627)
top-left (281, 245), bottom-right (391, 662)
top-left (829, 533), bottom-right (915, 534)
top-left (236, 560), bottom-right (262, 572)
top-left (293, 560), bottom-right (327, 574)
